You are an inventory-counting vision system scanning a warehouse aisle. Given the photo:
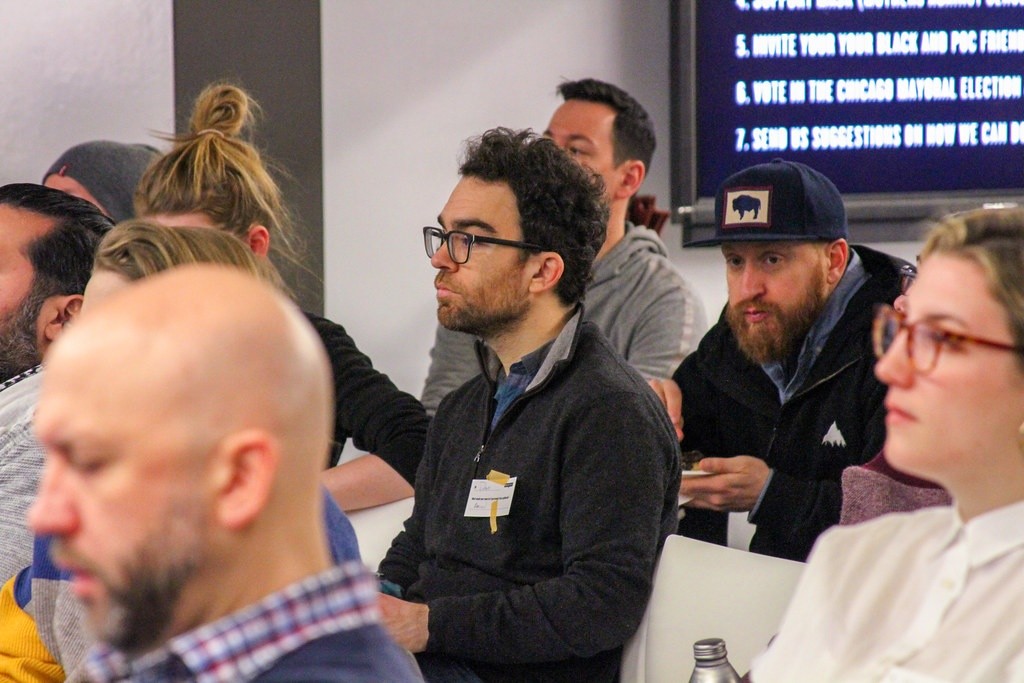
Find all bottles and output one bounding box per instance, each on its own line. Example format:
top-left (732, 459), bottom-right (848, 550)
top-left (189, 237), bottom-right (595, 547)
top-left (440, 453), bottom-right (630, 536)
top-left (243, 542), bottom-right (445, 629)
top-left (689, 638), bottom-right (741, 683)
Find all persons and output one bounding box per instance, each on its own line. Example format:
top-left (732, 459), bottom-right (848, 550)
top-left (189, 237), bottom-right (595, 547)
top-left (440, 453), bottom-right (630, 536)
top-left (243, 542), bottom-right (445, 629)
top-left (421, 76), bottom-right (706, 421)
top-left (730, 204), bottom-right (1024, 683)
top-left (369, 128), bottom-right (683, 680)
top-left (0, 81), bottom-right (433, 682)
top-left (643, 157), bottom-right (927, 560)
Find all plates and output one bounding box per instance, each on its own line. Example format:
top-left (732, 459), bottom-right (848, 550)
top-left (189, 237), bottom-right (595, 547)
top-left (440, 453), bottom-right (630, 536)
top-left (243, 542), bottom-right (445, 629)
top-left (682, 472), bottom-right (713, 478)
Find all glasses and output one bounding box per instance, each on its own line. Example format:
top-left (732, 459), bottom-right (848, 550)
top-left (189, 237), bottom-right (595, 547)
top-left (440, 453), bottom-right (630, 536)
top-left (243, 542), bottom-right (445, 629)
top-left (872, 304), bottom-right (1024, 375)
top-left (423, 227), bottom-right (543, 264)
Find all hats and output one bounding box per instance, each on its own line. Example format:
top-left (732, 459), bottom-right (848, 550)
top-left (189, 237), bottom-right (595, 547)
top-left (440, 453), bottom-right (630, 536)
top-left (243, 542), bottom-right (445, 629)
top-left (681, 158), bottom-right (848, 248)
top-left (43, 140), bottom-right (164, 224)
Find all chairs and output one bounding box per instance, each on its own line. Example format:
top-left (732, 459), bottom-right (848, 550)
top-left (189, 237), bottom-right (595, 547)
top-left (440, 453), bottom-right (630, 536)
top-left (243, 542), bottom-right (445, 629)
top-left (335, 439), bottom-right (413, 575)
top-left (617, 534), bottom-right (807, 682)
